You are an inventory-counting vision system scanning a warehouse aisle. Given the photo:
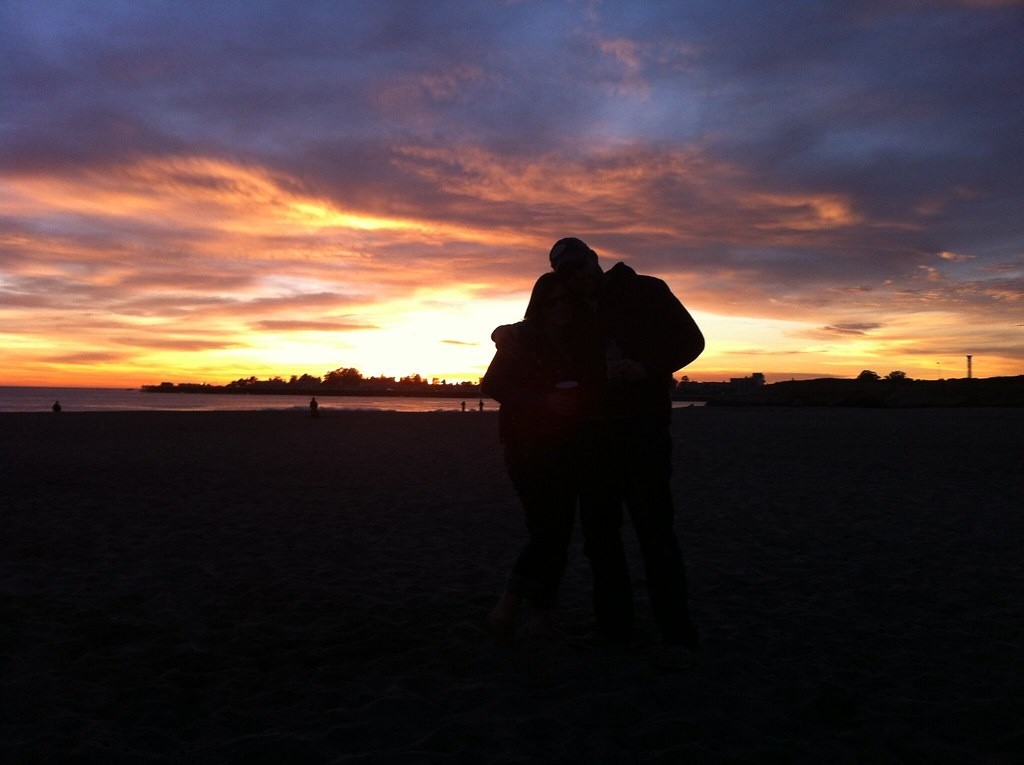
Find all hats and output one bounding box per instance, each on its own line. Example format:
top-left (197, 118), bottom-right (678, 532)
top-left (550, 237), bottom-right (590, 271)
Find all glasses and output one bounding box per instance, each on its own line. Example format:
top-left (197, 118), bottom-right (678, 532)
top-left (544, 294), bottom-right (571, 308)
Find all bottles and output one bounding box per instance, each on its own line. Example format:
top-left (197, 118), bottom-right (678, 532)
top-left (606, 339), bottom-right (624, 385)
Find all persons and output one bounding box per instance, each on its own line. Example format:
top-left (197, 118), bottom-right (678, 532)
top-left (310, 397), bottom-right (318, 415)
top-left (492, 239), bottom-right (707, 649)
top-left (480, 273), bottom-right (598, 646)
top-left (52, 401), bottom-right (62, 413)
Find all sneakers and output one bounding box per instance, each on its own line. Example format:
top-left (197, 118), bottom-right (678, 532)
top-left (528, 603), bottom-right (564, 639)
top-left (568, 629), bottom-right (636, 650)
top-left (658, 646), bottom-right (704, 670)
top-left (487, 592), bottom-right (522, 633)
